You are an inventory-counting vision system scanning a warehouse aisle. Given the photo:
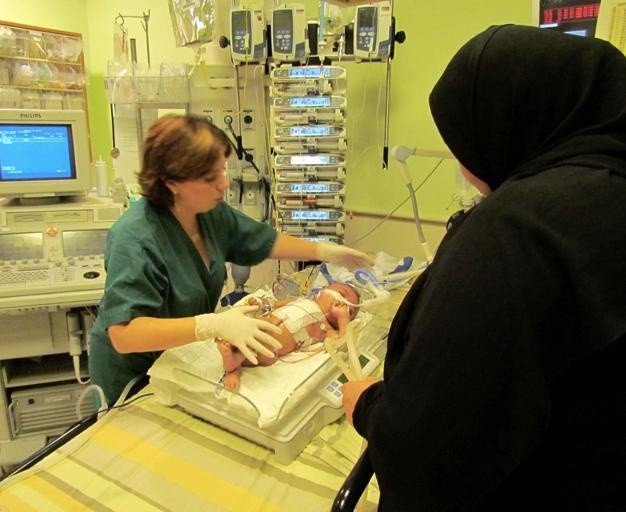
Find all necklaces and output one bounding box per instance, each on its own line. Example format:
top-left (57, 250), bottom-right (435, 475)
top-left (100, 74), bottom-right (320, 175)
top-left (173, 211), bottom-right (202, 243)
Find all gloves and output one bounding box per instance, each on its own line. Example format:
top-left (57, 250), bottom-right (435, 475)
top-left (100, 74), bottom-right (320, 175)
top-left (195, 305), bottom-right (283, 365)
top-left (316, 241), bottom-right (375, 271)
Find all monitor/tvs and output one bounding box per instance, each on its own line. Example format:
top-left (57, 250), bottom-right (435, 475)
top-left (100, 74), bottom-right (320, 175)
top-left (0, 111), bottom-right (91, 203)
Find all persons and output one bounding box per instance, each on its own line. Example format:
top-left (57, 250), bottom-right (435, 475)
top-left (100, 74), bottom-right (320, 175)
top-left (215, 282), bottom-right (359, 393)
top-left (89, 115), bottom-right (375, 412)
top-left (340, 23), bottom-right (626, 512)
